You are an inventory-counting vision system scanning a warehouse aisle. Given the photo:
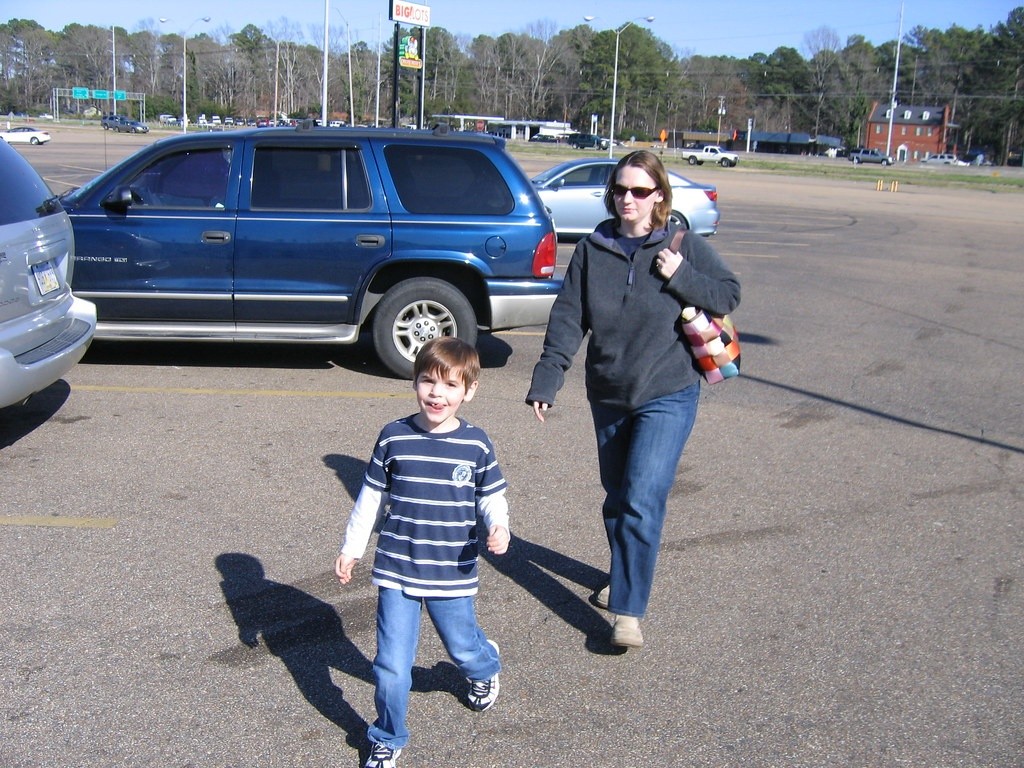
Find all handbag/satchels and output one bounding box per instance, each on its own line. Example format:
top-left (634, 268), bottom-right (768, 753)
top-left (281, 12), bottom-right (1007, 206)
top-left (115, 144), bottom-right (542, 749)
top-left (669, 229), bottom-right (741, 385)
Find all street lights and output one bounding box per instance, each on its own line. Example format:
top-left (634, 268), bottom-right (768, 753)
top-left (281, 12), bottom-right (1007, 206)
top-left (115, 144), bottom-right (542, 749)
top-left (159, 15), bottom-right (210, 132)
top-left (583, 16), bottom-right (656, 160)
top-left (717, 95), bottom-right (727, 145)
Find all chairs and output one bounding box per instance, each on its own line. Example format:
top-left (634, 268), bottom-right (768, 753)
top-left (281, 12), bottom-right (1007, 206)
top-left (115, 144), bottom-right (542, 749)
top-left (589, 167), bottom-right (606, 185)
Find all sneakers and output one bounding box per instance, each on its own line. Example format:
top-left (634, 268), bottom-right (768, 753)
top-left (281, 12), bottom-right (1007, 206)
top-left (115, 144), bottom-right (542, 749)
top-left (363, 741), bottom-right (402, 768)
top-left (467, 639), bottom-right (499, 712)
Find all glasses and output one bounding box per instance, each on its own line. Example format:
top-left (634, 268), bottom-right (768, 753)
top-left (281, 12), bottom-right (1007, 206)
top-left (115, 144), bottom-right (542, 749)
top-left (611, 184), bottom-right (660, 200)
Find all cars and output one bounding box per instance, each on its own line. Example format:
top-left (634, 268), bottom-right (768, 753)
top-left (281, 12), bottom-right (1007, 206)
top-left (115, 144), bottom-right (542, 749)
top-left (529, 158), bottom-right (720, 244)
top-left (112, 120), bottom-right (149, 134)
top-left (0, 126), bottom-right (51, 145)
top-left (0, 136), bottom-right (98, 409)
top-left (161, 113), bottom-right (507, 132)
top-left (528, 133), bottom-right (558, 144)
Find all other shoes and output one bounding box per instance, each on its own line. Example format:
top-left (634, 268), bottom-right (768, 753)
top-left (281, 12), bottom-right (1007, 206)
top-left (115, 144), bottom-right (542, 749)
top-left (609, 614), bottom-right (643, 648)
top-left (595, 585), bottom-right (611, 608)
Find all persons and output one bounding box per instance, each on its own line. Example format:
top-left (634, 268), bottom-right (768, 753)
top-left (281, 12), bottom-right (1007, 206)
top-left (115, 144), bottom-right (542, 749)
top-left (336, 336), bottom-right (510, 767)
top-left (977, 153), bottom-right (984, 166)
top-left (828, 148), bottom-right (836, 158)
top-left (144, 147), bottom-right (229, 205)
top-left (525, 150), bottom-right (741, 647)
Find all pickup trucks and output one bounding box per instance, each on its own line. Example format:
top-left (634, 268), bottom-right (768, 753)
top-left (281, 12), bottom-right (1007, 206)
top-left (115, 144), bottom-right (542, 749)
top-left (682, 145), bottom-right (739, 168)
top-left (848, 147), bottom-right (895, 167)
top-left (568, 132), bottom-right (610, 150)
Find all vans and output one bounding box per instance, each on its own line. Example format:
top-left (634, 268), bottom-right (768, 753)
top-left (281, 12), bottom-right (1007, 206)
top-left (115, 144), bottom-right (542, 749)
top-left (54, 119), bottom-right (567, 382)
top-left (921, 153), bottom-right (958, 166)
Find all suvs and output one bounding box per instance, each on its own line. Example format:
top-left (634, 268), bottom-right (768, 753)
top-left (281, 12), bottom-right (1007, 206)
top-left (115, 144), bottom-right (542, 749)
top-left (101, 115), bottom-right (127, 130)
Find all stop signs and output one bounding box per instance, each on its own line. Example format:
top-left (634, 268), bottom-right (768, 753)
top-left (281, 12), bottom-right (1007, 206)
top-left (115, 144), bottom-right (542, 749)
top-left (476, 120), bottom-right (485, 132)
